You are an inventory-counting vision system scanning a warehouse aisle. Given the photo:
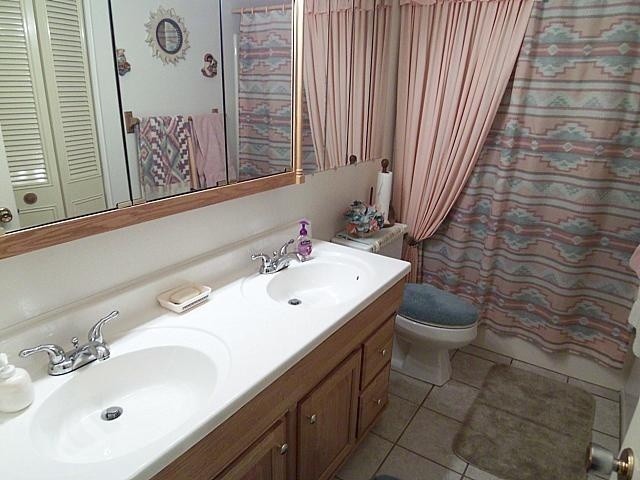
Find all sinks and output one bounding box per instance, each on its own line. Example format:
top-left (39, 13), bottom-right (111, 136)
top-left (29, 344), bottom-right (221, 466)
top-left (267, 260), bottom-right (369, 308)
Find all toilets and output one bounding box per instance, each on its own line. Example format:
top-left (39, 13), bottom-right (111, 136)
top-left (331, 221), bottom-right (479, 387)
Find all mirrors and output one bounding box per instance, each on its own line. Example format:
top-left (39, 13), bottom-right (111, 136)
top-left (145, 5), bottom-right (192, 65)
top-left (0, 1), bottom-right (303, 259)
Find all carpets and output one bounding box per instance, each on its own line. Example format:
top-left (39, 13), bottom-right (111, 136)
top-left (452, 363), bottom-right (596, 479)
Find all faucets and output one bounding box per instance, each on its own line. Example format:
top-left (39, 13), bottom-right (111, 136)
top-left (71, 341), bottom-right (110, 369)
top-left (276, 251), bottom-right (307, 270)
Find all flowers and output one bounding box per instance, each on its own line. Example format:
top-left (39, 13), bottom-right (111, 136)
top-left (339, 199), bottom-right (384, 234)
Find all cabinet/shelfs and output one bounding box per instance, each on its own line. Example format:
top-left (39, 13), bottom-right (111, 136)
top-left (214, 342), bottom-right (362, 480)
top-left (358, 280), bottom-right (405, 442)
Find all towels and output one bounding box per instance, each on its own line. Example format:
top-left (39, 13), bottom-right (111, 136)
top-left (136, 115), bottom-right (192, 200)
top-left (629, 245), bottom-right (640, 280)
top-left (628, 297), bottom-right (640, 359)
top-left (190, 113), bottom-right (230, 189)
